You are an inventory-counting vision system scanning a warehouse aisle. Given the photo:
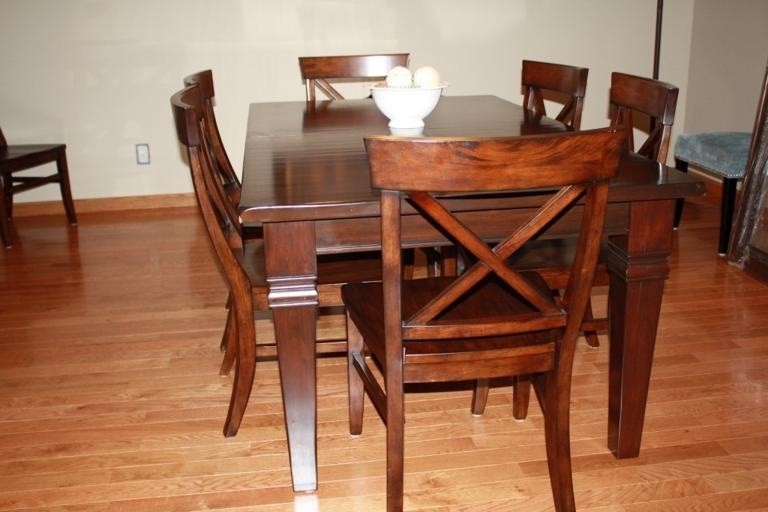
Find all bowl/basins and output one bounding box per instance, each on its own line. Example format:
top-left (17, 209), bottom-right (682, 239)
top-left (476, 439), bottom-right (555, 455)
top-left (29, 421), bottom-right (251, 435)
top-left (374, 81), bottom-right (445, 129)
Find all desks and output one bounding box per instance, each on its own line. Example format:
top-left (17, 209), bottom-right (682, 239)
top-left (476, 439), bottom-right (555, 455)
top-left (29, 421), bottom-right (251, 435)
top-left (239, 94), bottom-right (708, 493)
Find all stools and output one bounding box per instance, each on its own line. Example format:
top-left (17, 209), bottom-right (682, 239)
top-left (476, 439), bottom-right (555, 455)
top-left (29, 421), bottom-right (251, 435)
top-left (672, 132), bottom-right (752, 257)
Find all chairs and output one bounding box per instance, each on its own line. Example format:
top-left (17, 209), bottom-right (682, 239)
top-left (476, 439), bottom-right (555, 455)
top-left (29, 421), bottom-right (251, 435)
top-left (419, 60), bottom-right (599, 348)
top-left (298, 52), bottom-right (409, 102)
top-left (439, 72), bottom-right (680, 420)
top-left (340, 127), bottom-right (627, 512)
top-left (184, 69), bottom-right (264, 352)
top-left (0, 128), bottom-right (78, 248)
top-left (170, 86), bottom-right (413, 438)
